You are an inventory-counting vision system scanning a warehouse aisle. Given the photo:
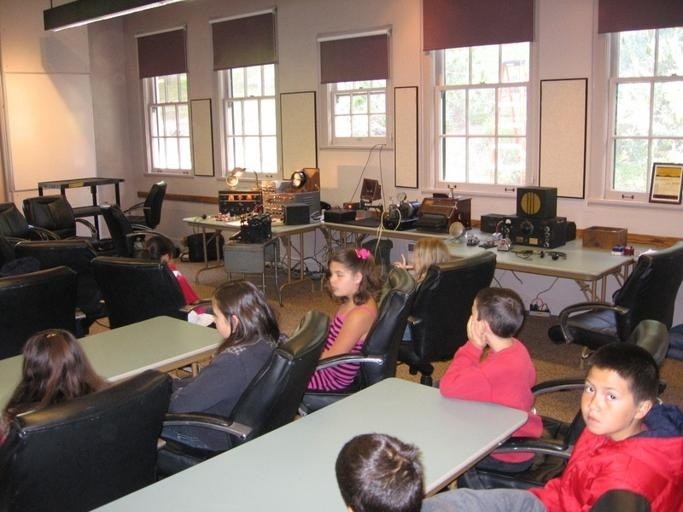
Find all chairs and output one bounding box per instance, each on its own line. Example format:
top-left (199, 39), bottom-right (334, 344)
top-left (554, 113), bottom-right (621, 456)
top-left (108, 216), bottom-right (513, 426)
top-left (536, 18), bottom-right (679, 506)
top-left (399, 251), bottom-right (496, 387)
top-left (159, 311), bottom-right (330, 476)
top-left (22, 196), bottom-right (98, 243)
top-left (1, 202), bottom-right (47, 257)
top-left (92, 257), bottom-right (213, 328)
top-left (1, 267), bottom-right (76, 359)
top-left (121, 181), bottom-right (167, 229)
top-left (99, 205), bottom-right (173, 256)
top-left (15, 238), bottom-right (109, 336)
top-left (300, 290), bottom-right (410, 417)
top-left (457, 375), bottom-right (666, 491)
top-left (1, 368), bottom-right (173, 508)
top-left (548, 240), bottom-right (682, 360)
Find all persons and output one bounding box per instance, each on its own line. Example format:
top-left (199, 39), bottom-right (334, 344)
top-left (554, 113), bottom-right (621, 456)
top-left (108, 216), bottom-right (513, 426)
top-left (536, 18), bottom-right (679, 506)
top-left (167, 278), bottom-right (289, 460)
top-left (138, 235), bottom-right (213, 316)
top-left (387, 237), bottom-right (450, 342)
top-left (525, 341), bottom-right (683, 512)
top-left (334, 432), bottom-right (547, 512)
top-left (438, 286), bottom-right (544, 490)
top-left (1, 328), bottom-right (118, 446)
top-left (304, 246), bottom-right (384, 391)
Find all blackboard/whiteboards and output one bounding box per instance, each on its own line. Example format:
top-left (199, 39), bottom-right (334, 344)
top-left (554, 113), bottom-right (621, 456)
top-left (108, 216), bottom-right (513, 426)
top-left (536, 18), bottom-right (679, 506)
top-left (4, 72), bottom-right (97, 192)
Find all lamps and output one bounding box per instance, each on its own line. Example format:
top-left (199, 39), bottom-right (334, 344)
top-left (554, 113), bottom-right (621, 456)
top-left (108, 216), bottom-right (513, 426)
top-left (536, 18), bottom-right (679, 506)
top-left (226, 167), bottom-right (258, 189)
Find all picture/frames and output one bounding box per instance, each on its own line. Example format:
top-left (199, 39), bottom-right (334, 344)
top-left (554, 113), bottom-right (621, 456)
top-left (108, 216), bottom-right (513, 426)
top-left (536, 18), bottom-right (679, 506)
top-left (189, 98), bottom-right (215, 177)
top-left (279, 91), bottom-right (318, 180)
top-left (648, 162), bottom-right (682, 203)
top-left (393, 86), bottom-right (419, 189)
top-left (539, 77), bottom-right (588, 198)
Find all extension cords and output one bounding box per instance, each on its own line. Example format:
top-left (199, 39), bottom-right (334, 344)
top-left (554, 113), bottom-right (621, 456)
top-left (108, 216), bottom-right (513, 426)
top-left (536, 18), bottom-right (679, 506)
top-left (528, 310), bottom-right (551, 318)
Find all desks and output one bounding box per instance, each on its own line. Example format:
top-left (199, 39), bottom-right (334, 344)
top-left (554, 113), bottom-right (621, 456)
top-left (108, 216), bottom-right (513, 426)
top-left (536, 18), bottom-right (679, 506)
top-left (321, 220), bottom-right (454, 292)
top-left (183, 211), bottom-right (321, 305)
top-left (87, 376), bottom-right (528, 511)
top-left (0, 315), bottom-right (223, 415)
top-left (38, 177), bottom-right (123, 236)
top-left (446, 227), bottom-right (633, 308)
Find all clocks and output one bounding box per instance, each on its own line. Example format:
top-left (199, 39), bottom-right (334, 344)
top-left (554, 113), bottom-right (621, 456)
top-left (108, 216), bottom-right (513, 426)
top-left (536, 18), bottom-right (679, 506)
top-left (290, 172), bottom-right (306, 187)
